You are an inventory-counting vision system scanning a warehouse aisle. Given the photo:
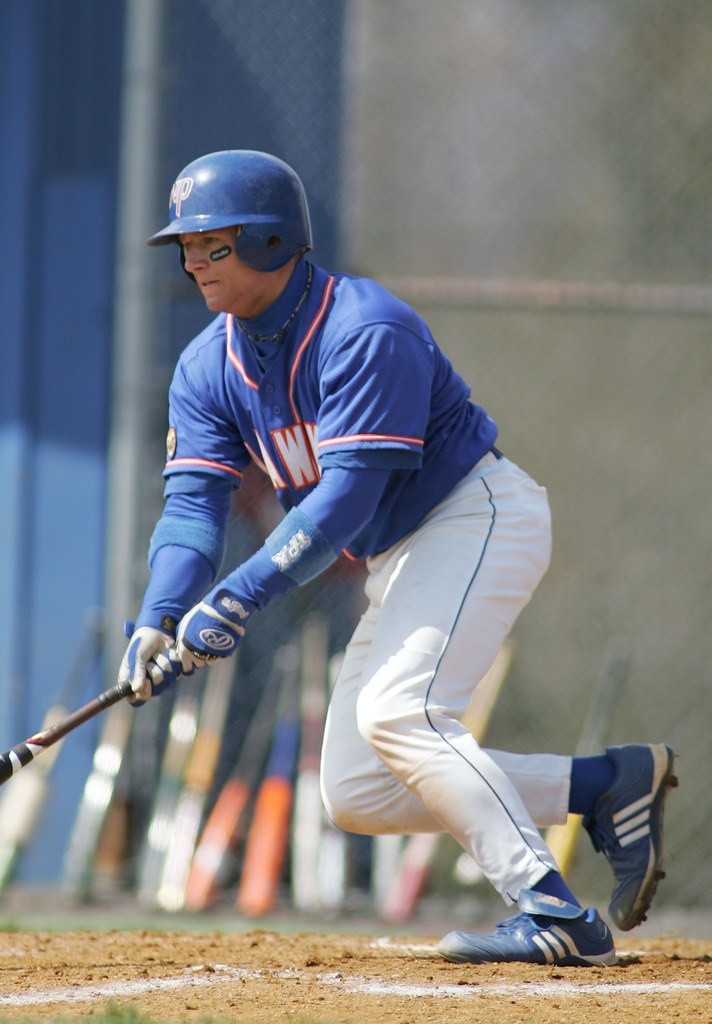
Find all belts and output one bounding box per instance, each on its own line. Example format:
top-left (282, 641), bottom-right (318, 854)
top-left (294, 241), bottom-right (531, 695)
top-left (470, 443), bottom-right (502, 474)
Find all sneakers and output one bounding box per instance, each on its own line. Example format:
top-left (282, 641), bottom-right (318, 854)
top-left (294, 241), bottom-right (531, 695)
top-left (438, 888), bottom-right (620, 967)
top-left (582, 743), bottom-right (680, 931)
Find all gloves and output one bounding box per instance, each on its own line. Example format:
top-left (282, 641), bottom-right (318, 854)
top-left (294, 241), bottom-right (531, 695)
top-left (118, 611), bottom-right (186, 708)
top-left (176, 587), bottom-right (255, 676)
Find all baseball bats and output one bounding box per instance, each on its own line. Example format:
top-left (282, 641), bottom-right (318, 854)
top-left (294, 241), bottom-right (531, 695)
top-left (0, 607), bottom-right (633, 923)
top-left (1, 675), bottom-right (130, 782)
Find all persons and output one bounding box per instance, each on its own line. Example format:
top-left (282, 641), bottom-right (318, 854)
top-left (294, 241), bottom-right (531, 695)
top-left (118, 149), bottom-right (678, 966)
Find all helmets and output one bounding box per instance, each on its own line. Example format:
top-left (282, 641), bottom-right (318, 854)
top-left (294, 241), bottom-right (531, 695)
top-left (147, 149), bottom-right (313, 272)
